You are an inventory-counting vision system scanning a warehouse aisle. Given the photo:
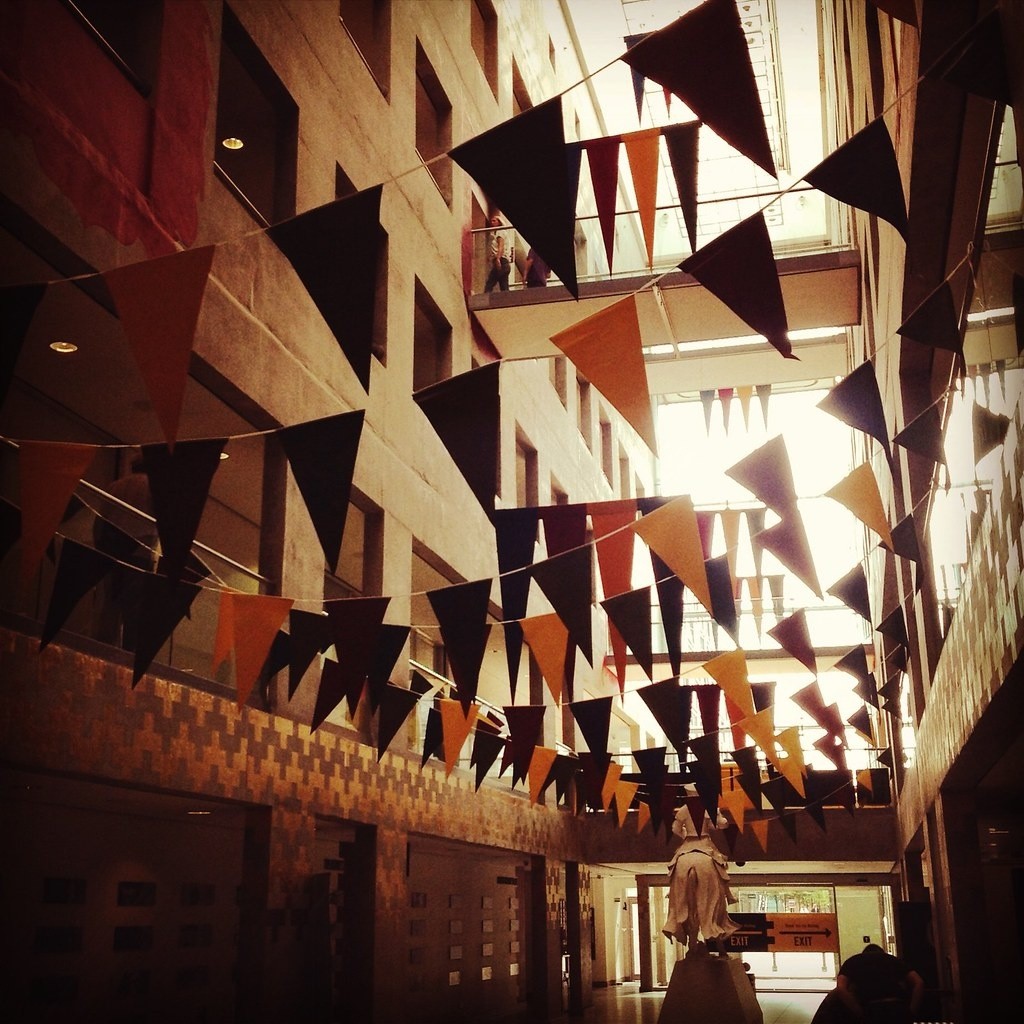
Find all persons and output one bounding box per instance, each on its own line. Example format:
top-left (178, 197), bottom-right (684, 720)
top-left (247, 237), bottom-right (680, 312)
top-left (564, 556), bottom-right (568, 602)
top-left (812, 943), bottom-right (926, 1024)
top-left (521, 246), bottom-right (553, 288)
top-left (662, 779), bottom-right (741, 906)
top-left (482, 215), bottom-right (515, 295)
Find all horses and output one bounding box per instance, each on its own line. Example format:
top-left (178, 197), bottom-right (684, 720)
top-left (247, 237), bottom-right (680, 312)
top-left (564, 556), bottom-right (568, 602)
top-left (662, 850), bottom-right (739, 957)
